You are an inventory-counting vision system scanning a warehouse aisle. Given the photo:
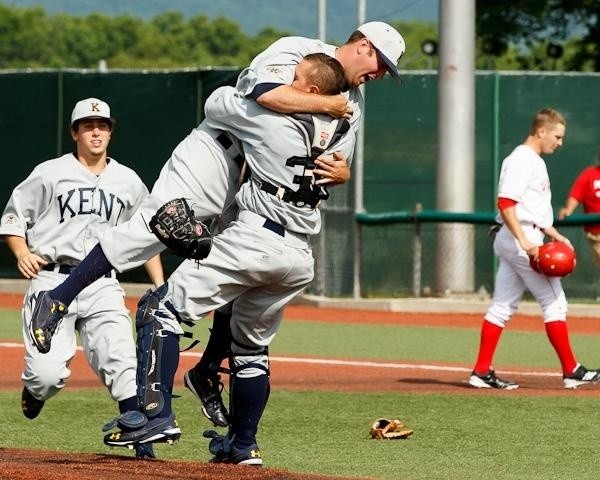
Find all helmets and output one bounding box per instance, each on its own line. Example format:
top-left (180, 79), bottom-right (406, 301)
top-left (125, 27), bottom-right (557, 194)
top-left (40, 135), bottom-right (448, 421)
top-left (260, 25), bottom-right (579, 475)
top-left (527, 241), bottom-right (576, 279)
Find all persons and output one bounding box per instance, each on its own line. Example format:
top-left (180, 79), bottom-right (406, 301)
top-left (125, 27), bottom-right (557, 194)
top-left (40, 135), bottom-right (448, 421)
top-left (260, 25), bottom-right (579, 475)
top-left (556, 147), bottom-right (600, 276)
top-left (103, 51), bottom-right (357, 467)
top-left (1, 96), bottom-right (168, 462)
top-left (466, 106), bottom-right (599, 391)
top-left (27, 17), bottom-right (407, 428)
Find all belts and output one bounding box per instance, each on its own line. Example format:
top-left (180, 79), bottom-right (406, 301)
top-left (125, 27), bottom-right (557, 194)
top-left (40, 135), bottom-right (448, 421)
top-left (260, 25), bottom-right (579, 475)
top-left (41, 262), bottom-right (116, 280)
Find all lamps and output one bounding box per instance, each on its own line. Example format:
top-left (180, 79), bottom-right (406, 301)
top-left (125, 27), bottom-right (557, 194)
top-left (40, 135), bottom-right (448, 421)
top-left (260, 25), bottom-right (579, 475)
top-left (421, 39), bottom-right (439, 57)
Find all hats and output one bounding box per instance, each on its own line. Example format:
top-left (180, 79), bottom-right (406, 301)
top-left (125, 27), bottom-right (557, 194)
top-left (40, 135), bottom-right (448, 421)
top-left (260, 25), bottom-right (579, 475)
top-left (353, 19), bottom-right (407, 87)
top-left (68, 96), bottom-right (116, 128)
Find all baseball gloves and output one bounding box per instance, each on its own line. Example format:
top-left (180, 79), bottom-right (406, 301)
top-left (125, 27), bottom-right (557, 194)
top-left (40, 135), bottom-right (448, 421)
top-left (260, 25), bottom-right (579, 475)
top-left (141, 198), bottom-right (213, 269)
top-left (371, 417), bottom-right (414, 439)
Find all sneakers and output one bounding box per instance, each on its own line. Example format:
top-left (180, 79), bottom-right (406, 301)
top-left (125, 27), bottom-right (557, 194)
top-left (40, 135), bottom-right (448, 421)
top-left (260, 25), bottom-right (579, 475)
top-left (104, 413), bottom-right (185, 449)
top-left (183, 365), bottom-right (229, 426)
top-left (205, 439), bottom-right (263, 466)
top-left (29, 290), bottom-right (70, 354)
top-left (560, 363), bottom-right (600, 390)
top-left (468, 368), bottom-right (520, 392)
top-left (18, 386), bottom-right (45, 422)
